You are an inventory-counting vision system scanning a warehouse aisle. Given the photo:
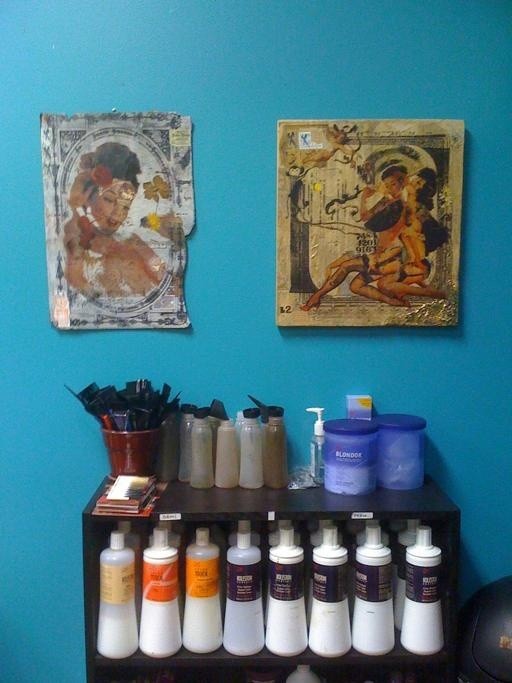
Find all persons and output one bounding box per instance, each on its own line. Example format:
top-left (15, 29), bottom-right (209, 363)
top-left (302, 131), bottom-right (361, 164)
top-left (62, 142), bottom-right (174, 300)
top-left (376, 166), bottom-right (450, 300)
top-left (299, 164), bottom-right (413, 312)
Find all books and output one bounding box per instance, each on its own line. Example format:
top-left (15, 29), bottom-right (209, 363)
top-left (90, 473), bottom-right (170, 516)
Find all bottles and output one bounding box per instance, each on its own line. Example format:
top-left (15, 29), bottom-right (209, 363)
top-left (153, 398), bottom-right (290, 488)
top-left (97, 518), bottom-right (448, 660)
top-left (323, 418), bottom-right (381, 498)
top-left (373, 413), bottom-right (428, 491)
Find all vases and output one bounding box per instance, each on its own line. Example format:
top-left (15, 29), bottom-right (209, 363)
top-left (100, 424), bottom-right (161, 479)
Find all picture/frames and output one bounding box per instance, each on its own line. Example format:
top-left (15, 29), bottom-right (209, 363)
top-left (276, 119), bottom-right (463, 328)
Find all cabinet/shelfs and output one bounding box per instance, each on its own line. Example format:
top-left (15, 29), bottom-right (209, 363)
top-left (82, 471), bottom-right (461, 682)
